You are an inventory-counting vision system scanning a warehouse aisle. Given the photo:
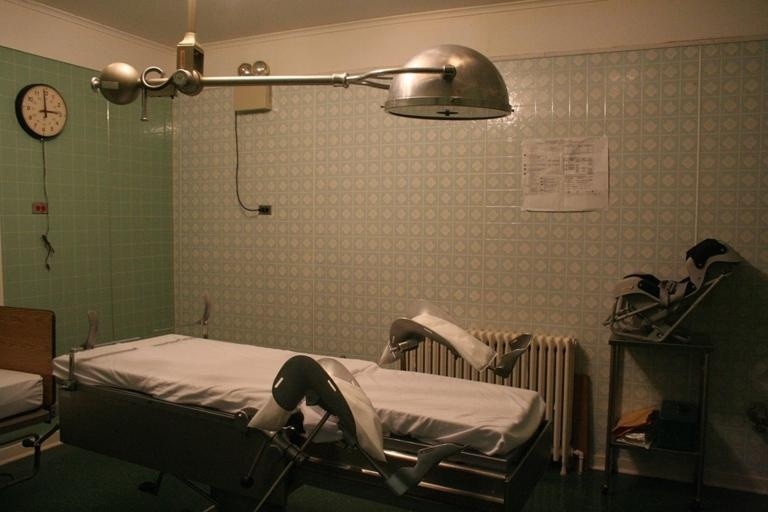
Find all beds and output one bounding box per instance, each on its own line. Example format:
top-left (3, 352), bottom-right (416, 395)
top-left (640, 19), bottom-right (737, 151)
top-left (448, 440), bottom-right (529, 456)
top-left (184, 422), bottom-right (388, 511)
top-left (50, 332), bottom-right (552, 505)
top-left (0, 308), bottom-right (56, 481)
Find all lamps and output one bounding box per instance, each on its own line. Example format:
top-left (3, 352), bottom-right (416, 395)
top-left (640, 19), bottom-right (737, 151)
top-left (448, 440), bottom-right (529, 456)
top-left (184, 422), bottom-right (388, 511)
top-left (235, 58), bottom-right (269, 76)
top-left (95, 1), bottom-right (513, 121)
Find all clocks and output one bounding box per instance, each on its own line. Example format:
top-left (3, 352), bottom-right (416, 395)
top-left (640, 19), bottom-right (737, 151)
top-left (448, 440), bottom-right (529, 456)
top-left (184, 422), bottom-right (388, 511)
top-left (17, 84), bottom-right (67, 141)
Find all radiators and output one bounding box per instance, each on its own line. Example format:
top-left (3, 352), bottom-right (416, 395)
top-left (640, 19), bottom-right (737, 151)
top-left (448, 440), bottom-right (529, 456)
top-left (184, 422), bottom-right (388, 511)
top-left (402, 330), bottom-right (585, 480)
